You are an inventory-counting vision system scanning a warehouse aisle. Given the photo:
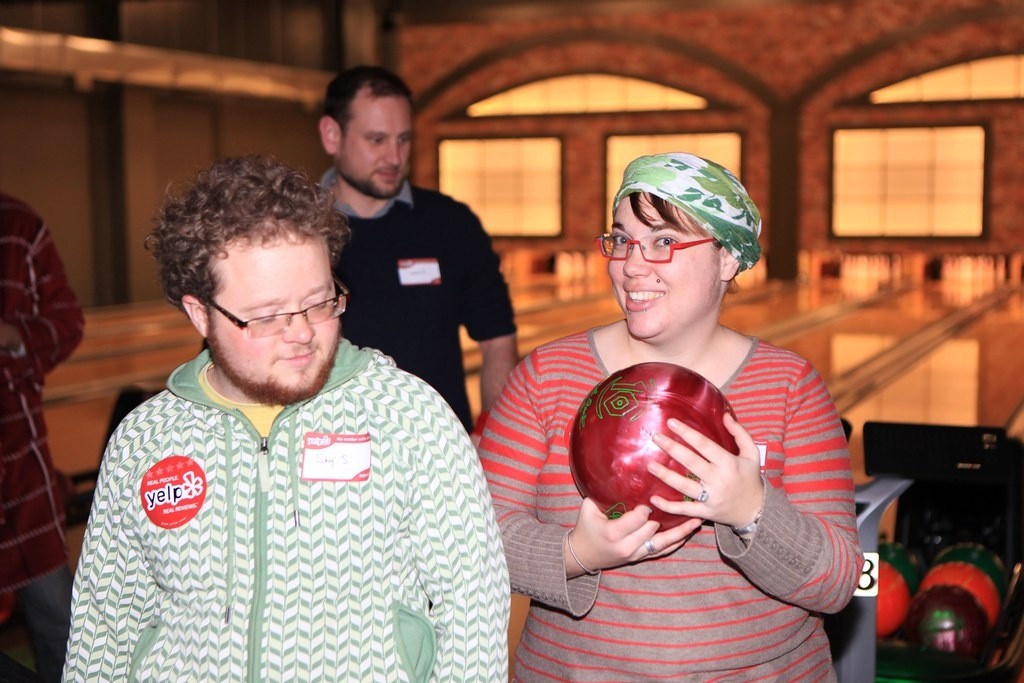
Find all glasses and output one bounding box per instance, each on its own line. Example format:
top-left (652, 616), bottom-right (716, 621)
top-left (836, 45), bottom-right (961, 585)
top-left (202, 279), bottom-right (348, 340)
top-left (593, 232), bottom-right (719, 265)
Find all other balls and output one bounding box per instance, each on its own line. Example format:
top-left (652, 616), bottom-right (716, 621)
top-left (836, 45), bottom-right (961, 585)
top-left (876, 542), bottom-right (1010, 657)
top-left (569, 360), bottom-right (743, 545)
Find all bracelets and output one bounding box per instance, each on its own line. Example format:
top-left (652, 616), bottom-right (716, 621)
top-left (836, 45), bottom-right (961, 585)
top-left (740, 535), bottom-right (754, 542)
top-left (731, 513), bottom-right (761, 535)
top-left (567, 532), bottom-right (599, 575)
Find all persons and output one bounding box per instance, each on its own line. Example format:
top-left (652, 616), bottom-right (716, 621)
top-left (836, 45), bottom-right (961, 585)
top-left (63, 152), bottom-right (509, 683)
top-left (315, 62), bottom-right (518, 436)
top-left (478, 151), bottom-right (864, 683)
top-left (0, 194), bottom-right (86, 683)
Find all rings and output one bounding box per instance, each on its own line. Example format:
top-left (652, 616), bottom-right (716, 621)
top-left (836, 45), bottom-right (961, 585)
top-left (698, 483), bottom-right (708, 502)
top-left (644, 541), bottom-right (656, 556)
